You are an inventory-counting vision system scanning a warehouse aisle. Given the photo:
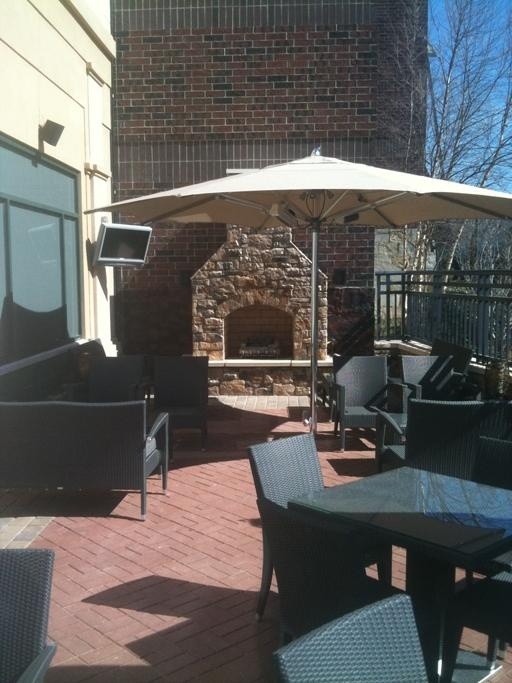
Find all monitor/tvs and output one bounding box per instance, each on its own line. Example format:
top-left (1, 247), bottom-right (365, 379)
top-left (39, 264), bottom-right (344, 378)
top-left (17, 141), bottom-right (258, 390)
top-left (91, 223), bottom-right (152, 268)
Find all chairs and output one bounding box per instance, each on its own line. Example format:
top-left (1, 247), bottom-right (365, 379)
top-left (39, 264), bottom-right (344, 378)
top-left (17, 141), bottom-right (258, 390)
top-left (334, 356), bottom-right (408, 452)
top-left (88, 354), bottom-right (209, 451)
top-left (1, 549), bottom-right (56, 683)
top-left (318, 353), bottom-right (341, 420)
top-left (1, 340), bottom-right (119, 423)
top-left (0, 399), bottom-right (169, 519)
top-left (383, 397), bottom-right (486, 487)
top-left (474, 400), bottom-right (512, 492)
top-left (271, 592), bottom-right (428, 683)
top-left (440, 576), bottom-right (512, 683)
top-left (247, 433), bottom-right (392, 622)
top-left (399, 353), bottom-right (465, 403)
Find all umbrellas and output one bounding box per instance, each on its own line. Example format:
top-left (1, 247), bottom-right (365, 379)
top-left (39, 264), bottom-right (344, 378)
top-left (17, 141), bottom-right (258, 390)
top-left (83, 149), bottom-right (512, 432)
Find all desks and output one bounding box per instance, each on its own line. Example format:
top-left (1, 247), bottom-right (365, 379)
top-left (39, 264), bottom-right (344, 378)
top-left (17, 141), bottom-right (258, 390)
top-left (288, 465), bottom-right (512, 682)
